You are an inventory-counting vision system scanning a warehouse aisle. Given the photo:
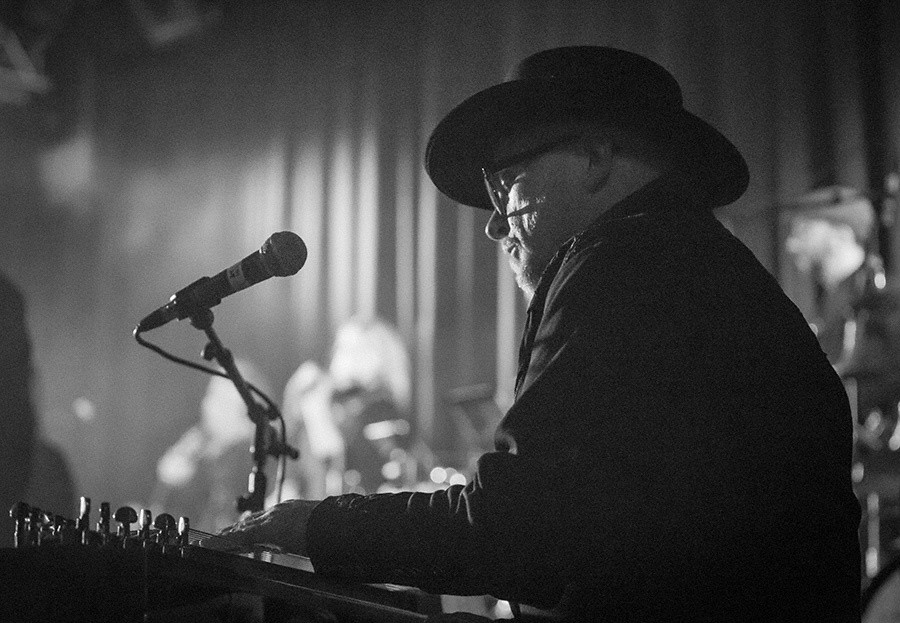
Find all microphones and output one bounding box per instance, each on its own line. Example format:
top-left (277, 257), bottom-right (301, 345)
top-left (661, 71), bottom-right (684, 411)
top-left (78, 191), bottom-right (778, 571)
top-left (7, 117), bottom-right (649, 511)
top-left (138, 231), bottom-right (307, 331)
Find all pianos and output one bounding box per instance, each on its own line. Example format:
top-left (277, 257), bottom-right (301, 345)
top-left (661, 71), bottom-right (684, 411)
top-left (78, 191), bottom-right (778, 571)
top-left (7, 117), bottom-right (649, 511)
top-left (0, 493), bottom-right (450, 623)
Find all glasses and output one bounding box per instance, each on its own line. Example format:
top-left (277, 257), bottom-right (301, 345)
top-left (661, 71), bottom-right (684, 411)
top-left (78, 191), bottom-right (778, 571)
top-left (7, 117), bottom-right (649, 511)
top-left (481, 132), bottom-right (620, 217)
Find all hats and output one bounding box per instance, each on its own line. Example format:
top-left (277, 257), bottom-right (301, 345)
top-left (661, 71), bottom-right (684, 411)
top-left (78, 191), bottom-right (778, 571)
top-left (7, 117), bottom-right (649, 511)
top-left (424, 46), bottom-right (750, 210)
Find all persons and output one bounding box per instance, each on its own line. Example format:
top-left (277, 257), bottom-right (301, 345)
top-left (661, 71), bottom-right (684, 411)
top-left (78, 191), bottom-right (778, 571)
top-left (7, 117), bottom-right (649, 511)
top-left (201, 44), bottom-right (860, 623)
